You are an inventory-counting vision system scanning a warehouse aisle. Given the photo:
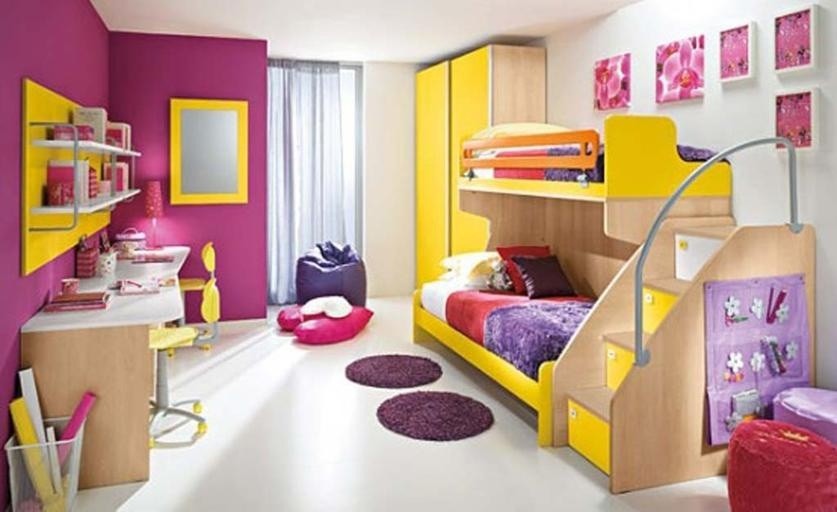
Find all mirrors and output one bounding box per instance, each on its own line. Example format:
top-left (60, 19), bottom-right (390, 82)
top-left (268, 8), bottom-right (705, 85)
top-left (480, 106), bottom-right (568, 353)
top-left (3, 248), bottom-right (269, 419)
top-left (168, 97), bottom-right (247, 205)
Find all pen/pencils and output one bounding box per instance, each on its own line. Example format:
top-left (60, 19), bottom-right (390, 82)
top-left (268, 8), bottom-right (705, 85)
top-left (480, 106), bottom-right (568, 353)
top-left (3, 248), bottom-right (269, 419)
top-left (99, 247), bottom-right (103, 255)
top-left (128, 281), bottom-right (142, 287)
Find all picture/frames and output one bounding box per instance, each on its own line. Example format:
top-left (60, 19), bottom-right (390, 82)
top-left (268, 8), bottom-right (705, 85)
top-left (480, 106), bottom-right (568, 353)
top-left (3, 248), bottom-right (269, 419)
top-left (720, 2), bottom-right (822, 151)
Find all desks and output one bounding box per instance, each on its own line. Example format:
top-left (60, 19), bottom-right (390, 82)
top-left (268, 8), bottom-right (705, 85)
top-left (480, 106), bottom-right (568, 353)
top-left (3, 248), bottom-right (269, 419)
top-left (22, 273), bottom-right (179, 489)
top-left (80, 246), bottom-right (190, 273)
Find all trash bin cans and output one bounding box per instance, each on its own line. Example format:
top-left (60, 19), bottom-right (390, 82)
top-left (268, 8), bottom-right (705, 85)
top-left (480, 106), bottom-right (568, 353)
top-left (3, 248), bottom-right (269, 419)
top-left (5, 416), bottom-right (87, 512)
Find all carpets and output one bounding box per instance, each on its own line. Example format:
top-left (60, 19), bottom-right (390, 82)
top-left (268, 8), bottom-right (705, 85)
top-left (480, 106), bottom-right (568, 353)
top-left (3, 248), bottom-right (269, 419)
top-left (346, 353), bottom-right (493, 441)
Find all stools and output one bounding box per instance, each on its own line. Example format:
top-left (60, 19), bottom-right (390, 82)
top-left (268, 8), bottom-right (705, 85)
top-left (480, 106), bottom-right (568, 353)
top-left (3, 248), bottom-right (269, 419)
top-left (728, 386), bottom-right (836, 512)
top-left (296, 251), bottom-right (366, 309)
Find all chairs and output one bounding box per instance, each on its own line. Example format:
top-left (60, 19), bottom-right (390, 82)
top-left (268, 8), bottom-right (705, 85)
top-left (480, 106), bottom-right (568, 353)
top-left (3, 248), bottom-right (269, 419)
top-left (149, 242), bottom-right (220, 444)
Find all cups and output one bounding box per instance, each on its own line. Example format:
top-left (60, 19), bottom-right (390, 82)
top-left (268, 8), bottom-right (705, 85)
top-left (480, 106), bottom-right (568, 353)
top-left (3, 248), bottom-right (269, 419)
top-left (97, 253), bottom-right (117, 277)
top-left (61, 277), bottom-right (78, 294)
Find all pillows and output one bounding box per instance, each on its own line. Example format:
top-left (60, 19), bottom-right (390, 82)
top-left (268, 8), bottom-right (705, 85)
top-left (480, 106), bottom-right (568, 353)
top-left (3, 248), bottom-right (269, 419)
top-left (441, 245), bottom-right (575, 301)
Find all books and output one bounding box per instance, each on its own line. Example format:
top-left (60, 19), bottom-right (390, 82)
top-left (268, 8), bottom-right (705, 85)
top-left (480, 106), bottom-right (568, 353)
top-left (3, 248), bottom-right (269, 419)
top-left (54, 107), bottom-right (132, 150)
top-left (45, 291), bottom-right (112, 312)
top-left (47, 159), bottom-right (130, 205)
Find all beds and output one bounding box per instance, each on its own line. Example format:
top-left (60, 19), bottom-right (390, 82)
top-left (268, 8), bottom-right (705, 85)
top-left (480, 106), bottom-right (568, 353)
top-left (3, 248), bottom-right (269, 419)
top-left (414, 120), bottom-right (733, 445)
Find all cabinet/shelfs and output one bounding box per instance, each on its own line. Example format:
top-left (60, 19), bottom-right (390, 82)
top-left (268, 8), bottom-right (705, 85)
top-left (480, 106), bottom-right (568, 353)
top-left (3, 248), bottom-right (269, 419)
top-left (413, 42), bottom-right (545, 291)
top-left (30, 112), bottom-right (141, 217)
